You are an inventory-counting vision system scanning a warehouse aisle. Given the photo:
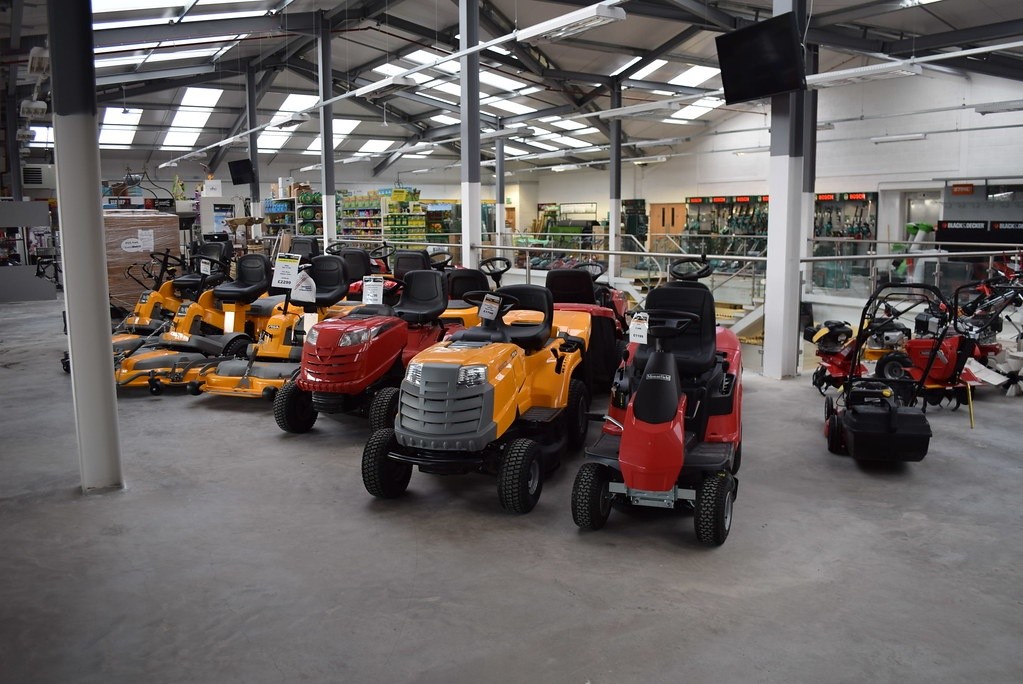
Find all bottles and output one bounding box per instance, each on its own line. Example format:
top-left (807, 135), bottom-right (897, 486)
top-left (384, 214), bottom-right (426, 239)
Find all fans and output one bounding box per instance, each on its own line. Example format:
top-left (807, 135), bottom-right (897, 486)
top-left (378, 101), bottom-right (407, 127)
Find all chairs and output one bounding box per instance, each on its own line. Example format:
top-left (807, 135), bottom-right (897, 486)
top-left (172, 242), bottom-right (716, 375)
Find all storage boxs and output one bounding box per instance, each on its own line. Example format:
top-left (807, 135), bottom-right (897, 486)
top-left (270, 176), bottom-right (312, 200)
top-left (842, 406), bottom-right (932, 462)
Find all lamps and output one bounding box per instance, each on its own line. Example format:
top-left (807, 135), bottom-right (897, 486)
top-left (16, 48), bottom-right (50, 157)
top-left (599, 103), bottom-right (680, 119)
top-left (870, 133), bottom-right (926, 143)
top-left (300, 166), bottom-right (322, 172)
top-left (806, 63), bottom-right (923, 91)
top-left (271, 112), bottom-right (310, 129)
top-left (356, 77), bottom-right (417, 99)
top-left (480, 127), bottom-right (535, 141)
top-left (398, 143), bottom-right (438, 153)
top-left (159, 162), bottom-right (178, 169)
top-left (184, 151), bottom-right (207, 160)
top-left (220, 137), bottom-right (248, 147)
top-left (412, 138), bottom-right (690, 178)
top-left (517, 4), bottom-right (627, 44)
top-left (815, 122), bottom-right (834, 131)
top-left (975, 100), bottom-right (1023, 115)
top-left (342, 156), bottom-right (370, 164)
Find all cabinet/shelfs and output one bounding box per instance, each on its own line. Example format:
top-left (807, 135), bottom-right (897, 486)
top-left (265, 188), bottom-right (463, 251)
